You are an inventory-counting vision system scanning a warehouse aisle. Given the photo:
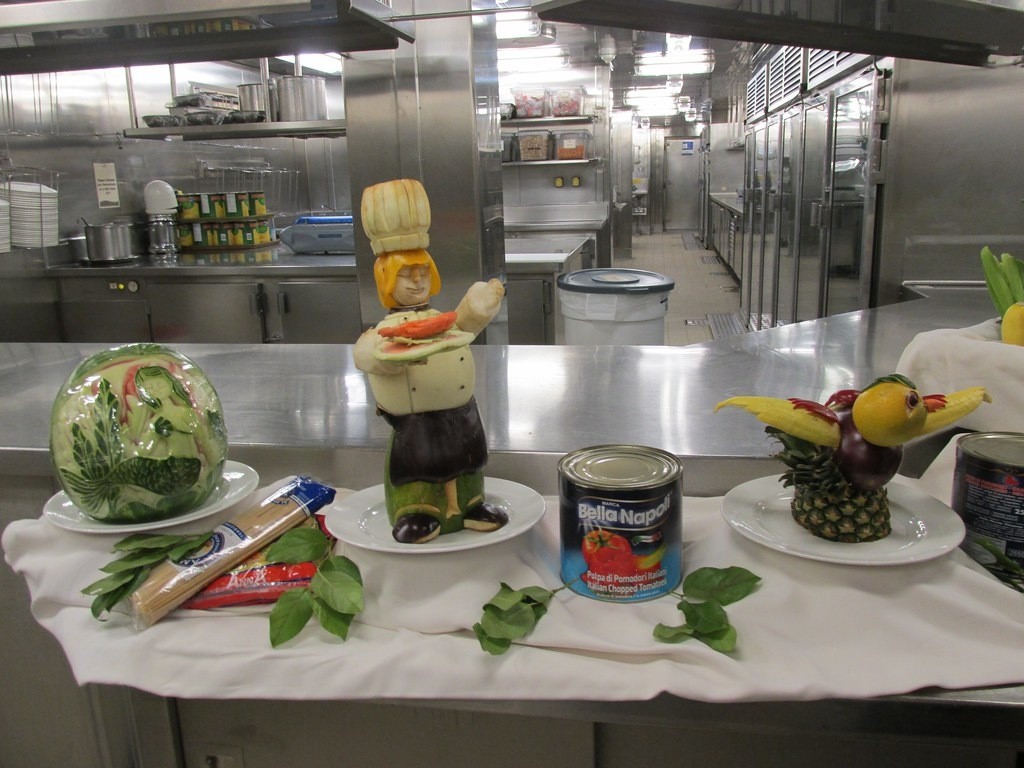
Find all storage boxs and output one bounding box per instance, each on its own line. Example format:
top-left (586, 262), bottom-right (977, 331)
top-left (515, 129), bottom-right (554, 161)
top-left (500, 85), bottom-right (588, 119)
top-left (501, 132), bottom-right (517, 161)
top-left (552, 129), bottom-right (592, 160)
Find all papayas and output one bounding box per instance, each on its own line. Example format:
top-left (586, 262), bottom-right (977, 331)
top-left (384, 427), bottom-right (486, 535)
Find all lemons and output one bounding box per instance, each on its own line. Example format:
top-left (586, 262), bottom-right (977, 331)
top-left (1000, 301), bottom-right (1024, 345)
top-left (853, 382), bottom-right (927, 448)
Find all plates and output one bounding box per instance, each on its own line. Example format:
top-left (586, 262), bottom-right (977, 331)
top-left (43, 455), bottom-right (259, 536)
top-left (722, 473), bottom-right (966, 566)
top-left (0, 181), bottom-right (59, 254)
top-left (325, 476), bottom-right (546, 552)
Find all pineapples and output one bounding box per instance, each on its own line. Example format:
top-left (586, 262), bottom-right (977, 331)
top-left (760, 426), bottom-right (892, 545)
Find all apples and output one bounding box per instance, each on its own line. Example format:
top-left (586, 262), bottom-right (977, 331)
top-left (834, 411), bottom-right (904, 491)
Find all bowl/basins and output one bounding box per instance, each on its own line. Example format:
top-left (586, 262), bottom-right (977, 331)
top-left (184, 111), bottom-right (227, 126)
top-left (141, 114), bottom-right (182, 128)
top-left (228, 109), bottom-right (266, 123)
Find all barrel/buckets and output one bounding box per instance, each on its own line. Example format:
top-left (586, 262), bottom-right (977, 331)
top-left (556, 267), bottom-right (675, 345)
top-left (238, 75), bottom-right (328, 123)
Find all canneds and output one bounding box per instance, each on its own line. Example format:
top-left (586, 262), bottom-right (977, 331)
top-left (555, 444), bottom-right (684, 604)
top-left (176, 189), bottom-right (270, 247)
top-left (950, 431), bottom-right (1024, 582)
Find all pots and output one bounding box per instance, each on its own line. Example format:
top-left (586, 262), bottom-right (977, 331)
top-left (84, 220), bottom-right (141, 261)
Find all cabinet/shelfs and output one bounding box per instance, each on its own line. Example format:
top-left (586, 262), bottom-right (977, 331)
top-left (709, 192), bottom-right (744, 280)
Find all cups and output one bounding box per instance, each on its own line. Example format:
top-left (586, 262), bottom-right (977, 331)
top-left (149, 213), bottom-right (178, 253)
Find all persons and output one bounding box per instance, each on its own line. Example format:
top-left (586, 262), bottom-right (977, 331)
top-left (352, 179), bottom-right (508, 545)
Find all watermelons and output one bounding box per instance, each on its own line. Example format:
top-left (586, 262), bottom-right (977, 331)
top-left (49, 343), bottom-right (228, 523)
top-left (377, 311), bottom-right (458, 340)
